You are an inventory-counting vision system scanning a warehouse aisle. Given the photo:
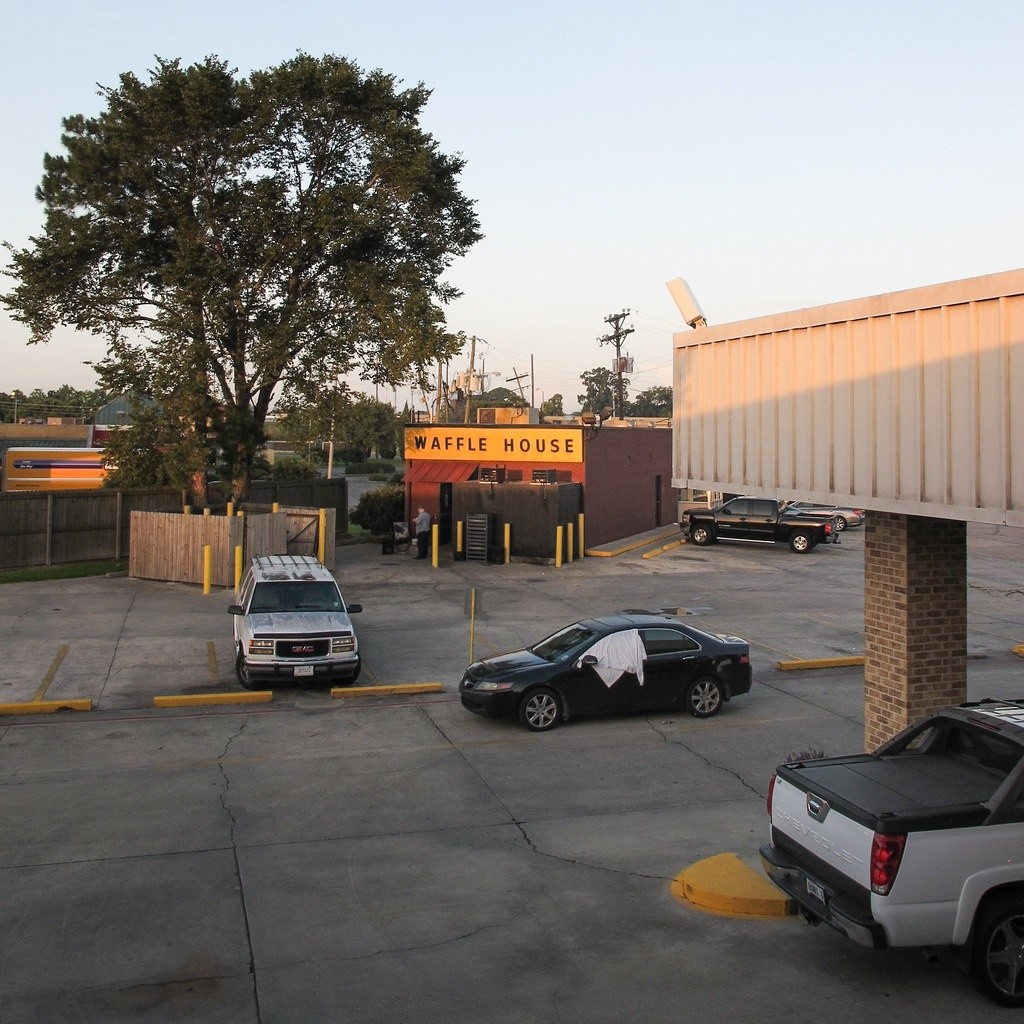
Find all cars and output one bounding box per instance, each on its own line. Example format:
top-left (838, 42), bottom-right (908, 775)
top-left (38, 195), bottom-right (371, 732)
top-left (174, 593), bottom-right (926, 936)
top-left (760, 697), bottom-right (1023, 1008)
top-left (24, 417), bottom-right (36, 425)
top-left (788, 501), bottom-right (864, 531)
top-left (458, 614), bottom-right (753, 731)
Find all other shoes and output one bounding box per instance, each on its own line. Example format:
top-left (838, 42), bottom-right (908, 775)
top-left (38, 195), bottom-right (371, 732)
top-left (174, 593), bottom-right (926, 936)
top-left (413, 556), bottom-right (427, 559)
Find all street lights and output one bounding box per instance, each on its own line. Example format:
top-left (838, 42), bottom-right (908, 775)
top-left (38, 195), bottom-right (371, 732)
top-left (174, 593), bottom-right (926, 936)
top-left (368, 358), bottom-right (379, 459)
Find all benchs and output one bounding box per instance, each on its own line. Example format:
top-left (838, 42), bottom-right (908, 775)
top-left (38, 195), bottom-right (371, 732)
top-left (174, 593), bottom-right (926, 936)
top-left (783, 509), bottom-right (802, 516)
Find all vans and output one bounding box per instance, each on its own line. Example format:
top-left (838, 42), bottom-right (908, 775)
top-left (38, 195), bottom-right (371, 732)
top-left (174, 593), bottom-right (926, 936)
top-left (228, 554), bottom-right (362, 690)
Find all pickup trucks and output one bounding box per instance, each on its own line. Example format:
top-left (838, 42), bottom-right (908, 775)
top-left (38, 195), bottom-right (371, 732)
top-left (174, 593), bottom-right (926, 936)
top-left (679, 496), bottom-right (839, 553)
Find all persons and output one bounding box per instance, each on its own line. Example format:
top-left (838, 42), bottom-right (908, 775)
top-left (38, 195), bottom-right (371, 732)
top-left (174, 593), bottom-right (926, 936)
top-left (412, 506), bottom-right (430, 560)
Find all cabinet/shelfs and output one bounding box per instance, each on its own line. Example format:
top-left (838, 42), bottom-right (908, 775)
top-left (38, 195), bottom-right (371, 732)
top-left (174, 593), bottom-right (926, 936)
top-left (466, 512), bottom-right (494, 563)
